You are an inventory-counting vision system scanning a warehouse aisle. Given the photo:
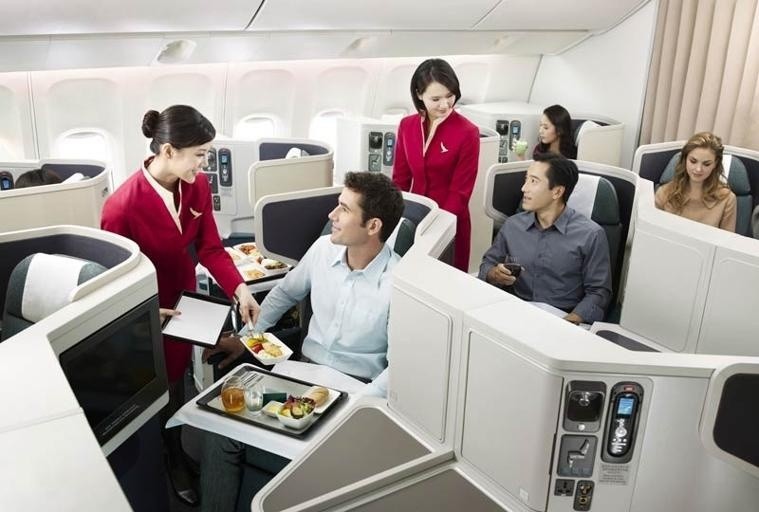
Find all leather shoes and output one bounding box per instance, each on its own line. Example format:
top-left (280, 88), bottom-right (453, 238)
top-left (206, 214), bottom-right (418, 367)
top-left (166, 452), bottom-right (200, 507)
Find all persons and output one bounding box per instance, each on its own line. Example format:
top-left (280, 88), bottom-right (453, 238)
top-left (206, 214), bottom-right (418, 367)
top-left (510, 104), bottom-right (577, 160)
top-left (654, 132), bottom-right (737, 232)
top-left (390, 58), bottom-right (481, 274)
top-left (197, 172), bottom-right (406, 512)
top-left (101, 104), bottom-right (260, 512)
top-left (479, 152), bottom-right (614, 327)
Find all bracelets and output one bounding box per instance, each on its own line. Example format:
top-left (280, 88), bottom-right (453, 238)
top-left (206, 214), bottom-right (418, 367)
top-left (568, 320), bottom-right (579, 325)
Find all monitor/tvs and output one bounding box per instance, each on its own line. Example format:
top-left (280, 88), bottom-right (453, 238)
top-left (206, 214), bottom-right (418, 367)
top-left (59, 294), bottom-right (169, 444)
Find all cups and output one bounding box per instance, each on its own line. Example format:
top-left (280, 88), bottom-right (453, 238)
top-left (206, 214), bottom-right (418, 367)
top-left (502, 254), bottom-right (522, 277)
top-left (220, 377), bottom-right (244, 413)
top-left (242, 382), bottom-right (265, 416)
top-left (515, 141), bottom-right (527, 154)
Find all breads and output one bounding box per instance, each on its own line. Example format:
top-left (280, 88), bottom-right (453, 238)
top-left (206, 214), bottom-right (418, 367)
top-left (310, 388), bottom-right (329, 407)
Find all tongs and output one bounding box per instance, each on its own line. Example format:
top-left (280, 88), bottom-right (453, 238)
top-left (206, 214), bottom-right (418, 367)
top-left (240, 303), bottom-right (265, 341)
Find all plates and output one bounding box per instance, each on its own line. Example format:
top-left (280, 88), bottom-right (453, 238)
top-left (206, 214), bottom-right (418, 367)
top-left (223, 242), bottom-right (293, 282)
top-left (261, 386), bottom-right (342, 429)
top-left (239, 331), bottom-right (294, 365)
